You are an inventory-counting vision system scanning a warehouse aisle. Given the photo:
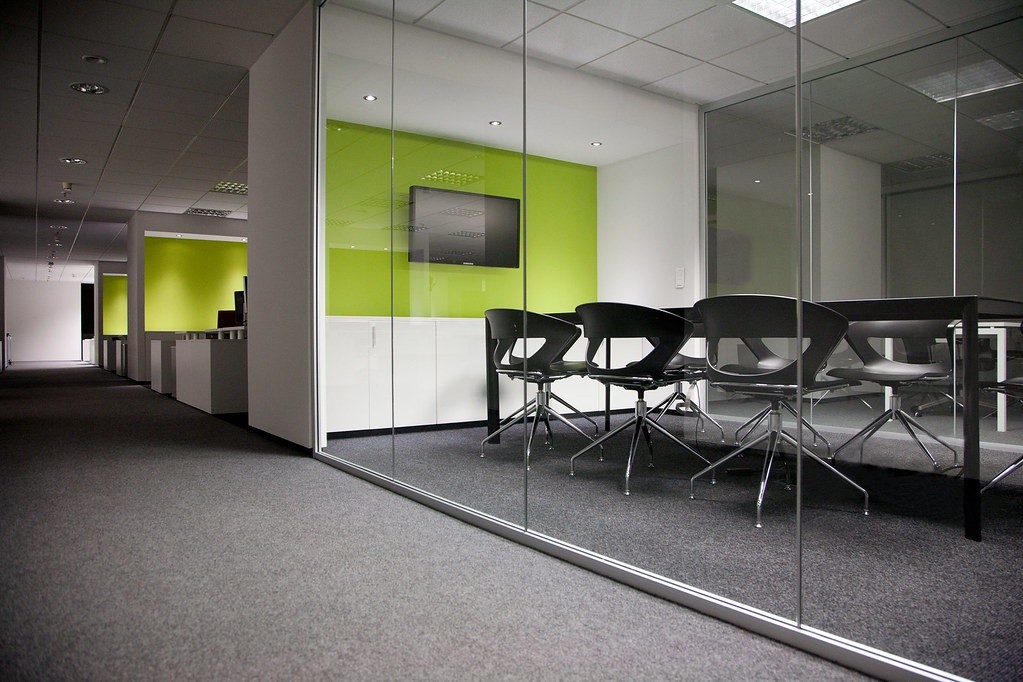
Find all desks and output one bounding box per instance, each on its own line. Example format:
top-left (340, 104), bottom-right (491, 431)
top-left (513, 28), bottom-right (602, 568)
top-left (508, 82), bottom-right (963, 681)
top-left (886, 321), bottom-right (1021, 433)
top-left (486, 295), bottom-right (1023, 540)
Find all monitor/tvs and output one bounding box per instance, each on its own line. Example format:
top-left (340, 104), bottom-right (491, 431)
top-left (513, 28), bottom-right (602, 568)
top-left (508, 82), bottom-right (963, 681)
top-left (407, 185), bottom-right (521, 269)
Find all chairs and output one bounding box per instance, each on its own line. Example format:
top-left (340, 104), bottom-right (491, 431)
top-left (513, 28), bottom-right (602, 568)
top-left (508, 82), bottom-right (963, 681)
top-left (716, 339), bottom-right (832, 461)
top-left (624, 336), bottom-right (725, 439)
top-left (571, 302), bottom-right (716, 504)
top-left (832, 318), bottom-right (964, 469)
top-left (688, 293), bottom-right (870, 524)
top-left (480, 308), bottom-right (604, 470)
